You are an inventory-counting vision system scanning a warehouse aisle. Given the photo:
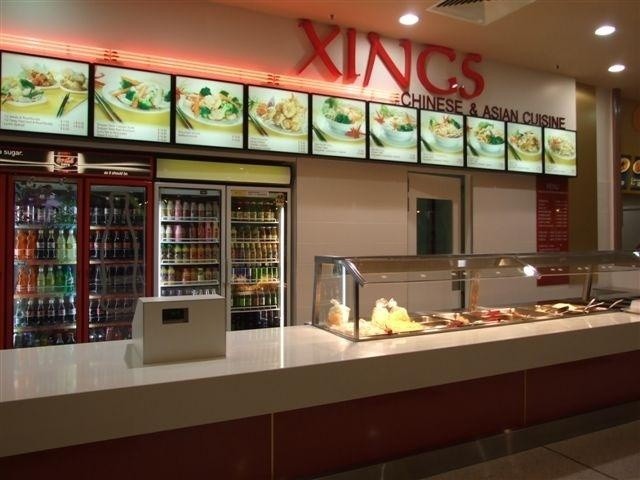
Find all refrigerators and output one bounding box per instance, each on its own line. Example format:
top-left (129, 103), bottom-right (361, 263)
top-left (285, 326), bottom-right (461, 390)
top-left (155, 157), bottom-right (293, 331)
top-left (0, 146), bottom-right (154, 350)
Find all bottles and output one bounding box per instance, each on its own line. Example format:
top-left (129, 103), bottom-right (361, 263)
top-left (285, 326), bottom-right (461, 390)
top-left (89, 196), bottom-right (143, 340)
top-left (13, 191), bottom-right (77, 347)
top-left (161, 197), bottom-right (219, 295)
top-left (230, 200), bottom-right (280, 328)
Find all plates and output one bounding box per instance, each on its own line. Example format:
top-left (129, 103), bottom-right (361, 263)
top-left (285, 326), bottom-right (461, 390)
top-left (424, 131), bottom-right (462, 153)
top-left (620, 157), bottom-right (630, 173)
top-left (249, 100), bottom-right (308, 137)
top-left (633, 159), bottom-right (640, 174)
top-left (372, 122), bottom-right (416, 149)
top-left (508, 134), bottom-right (542, 156)
top-left (0, 77), bottom-right (48, 108)
top-left (97, 82), bottom-right (106, 90)
top-left (177, 90), bottom-right (243, 127)
top-left (313, 111), bottom-right (366, 142)
top-left (19, 71), bottom-right (59, 90)
top-left (57, 75), bottom-right (90, 93)
top-left (544, 138), bottom-right (575, 159)
top-left (470, 131), bottom-right (505, 156)
top-left (103, 80), bottom-right (170, 113)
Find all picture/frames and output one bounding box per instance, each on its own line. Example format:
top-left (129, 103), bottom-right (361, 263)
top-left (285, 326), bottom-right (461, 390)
top-left (466, 115), bottom-right (507, 171)
top-left (544, 126), bottom-right (578, 177)
top-left (419, 108), bottom-right (465, 168)
top-left (92, 62), bottom-right (173, 146)
top-left (369, 100), bottom-right (420, 163)
top-left (312, 93), bottom-right (368, 158)
top-left (174, 75), bottom-right (247, 152)
top-left (248, 85), bottom-right (311, 155)
top-left (0, 51), bottom-right (90, 139)
top-left (507, 121), bottom-right (544, 175)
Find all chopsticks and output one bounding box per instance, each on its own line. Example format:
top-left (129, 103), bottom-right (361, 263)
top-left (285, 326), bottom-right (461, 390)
top-left (176, 105), bottom-right (194, 131)
top-left (248, 112), bottom-right (269, 136)
top-left (467, 142), bottom-right (480, 156)
top-left (55, 92), bottom-right (70, 118)
top-left (508, 142), bottom-right (520, 161)
top-left (96, 93), bottom-right (122, 123)
top-left (544, 147), bottom-right (555, 164)
top-left (311, 124), bottom-right (327, 141)
top-left (370, 131), bottom-right (384, 148)
top-left (420, 136), bottom-right (433, 153)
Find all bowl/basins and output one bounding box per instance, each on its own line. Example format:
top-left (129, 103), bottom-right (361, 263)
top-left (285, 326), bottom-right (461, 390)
top-left (323, 114), bottom-right (363, 135)
top-left (477, 138), bottom-right (503, 153)
top-left (378, 121), bottom-right (414, 142)
top-left (432, 130), bottom-right (462, 148)
